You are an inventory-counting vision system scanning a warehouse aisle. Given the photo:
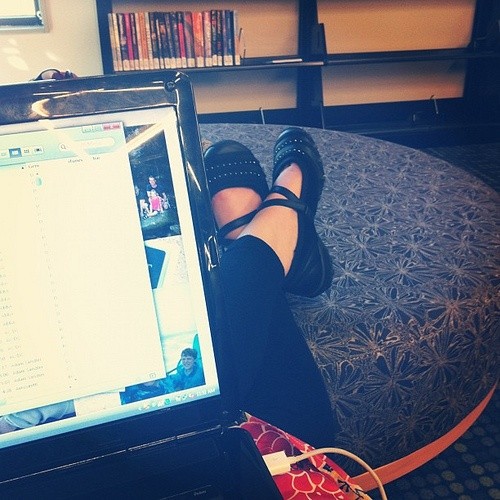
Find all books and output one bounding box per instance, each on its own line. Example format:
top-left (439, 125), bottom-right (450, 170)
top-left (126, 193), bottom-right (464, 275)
top-left (105, 8), bottom-right (242, 73)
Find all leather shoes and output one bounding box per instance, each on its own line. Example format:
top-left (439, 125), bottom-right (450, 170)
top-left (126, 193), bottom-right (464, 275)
top-left (257, 129), bottom-right (334, 297)
top-left (203, 140), bottom-right (269, 243)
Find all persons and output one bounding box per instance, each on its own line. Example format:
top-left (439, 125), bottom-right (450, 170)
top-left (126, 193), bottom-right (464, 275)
top-left (174, 347), bottom-right (205, 385)
top-left (138, 177), bottom-right (171, 218)
top-left (202, 126), bottom-right (372, 500)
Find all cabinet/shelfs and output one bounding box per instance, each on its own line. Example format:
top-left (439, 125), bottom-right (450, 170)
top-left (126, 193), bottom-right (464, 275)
top-left (96, 0), bottom-right (500, 147)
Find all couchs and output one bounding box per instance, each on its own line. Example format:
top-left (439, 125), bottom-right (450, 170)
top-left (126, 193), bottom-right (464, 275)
top-left (198, 121), bottom-right (500, 491)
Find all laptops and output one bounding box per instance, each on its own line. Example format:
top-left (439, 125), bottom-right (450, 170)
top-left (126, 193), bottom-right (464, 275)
top-left (0, 69), bottom-right (283, 500)
